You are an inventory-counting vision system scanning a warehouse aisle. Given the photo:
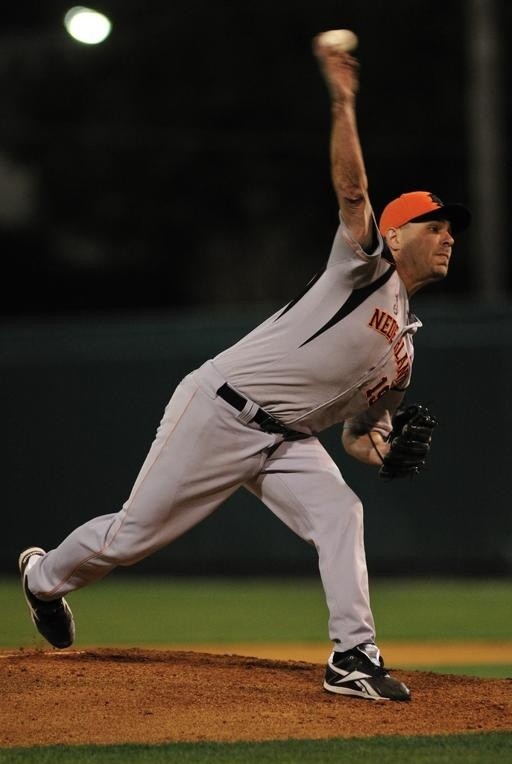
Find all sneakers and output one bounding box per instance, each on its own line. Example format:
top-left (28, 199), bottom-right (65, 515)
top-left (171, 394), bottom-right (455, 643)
top-left (323, 643), bottom-right (411, 701)
top-left (18, 547), bottom-right (75, 649)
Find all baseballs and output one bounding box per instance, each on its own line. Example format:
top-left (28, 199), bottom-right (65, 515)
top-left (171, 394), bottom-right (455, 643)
top-left (322, 29), bottom-right (357, 54)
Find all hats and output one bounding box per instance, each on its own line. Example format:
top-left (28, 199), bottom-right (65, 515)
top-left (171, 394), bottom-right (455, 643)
top-left (379, 191), bottom-right (472, 238)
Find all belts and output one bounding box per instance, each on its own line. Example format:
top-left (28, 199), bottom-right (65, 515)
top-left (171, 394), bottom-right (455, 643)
top-left (216, 382), bottom-right (303, 434)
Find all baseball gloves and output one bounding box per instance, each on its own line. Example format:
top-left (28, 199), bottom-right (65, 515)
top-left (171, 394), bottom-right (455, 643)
top-left (378, 404), bottom-right (438, 480)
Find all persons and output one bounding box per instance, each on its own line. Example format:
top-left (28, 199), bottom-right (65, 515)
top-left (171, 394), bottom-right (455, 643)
top-left (16, 27), bottom-right (457, 703)
